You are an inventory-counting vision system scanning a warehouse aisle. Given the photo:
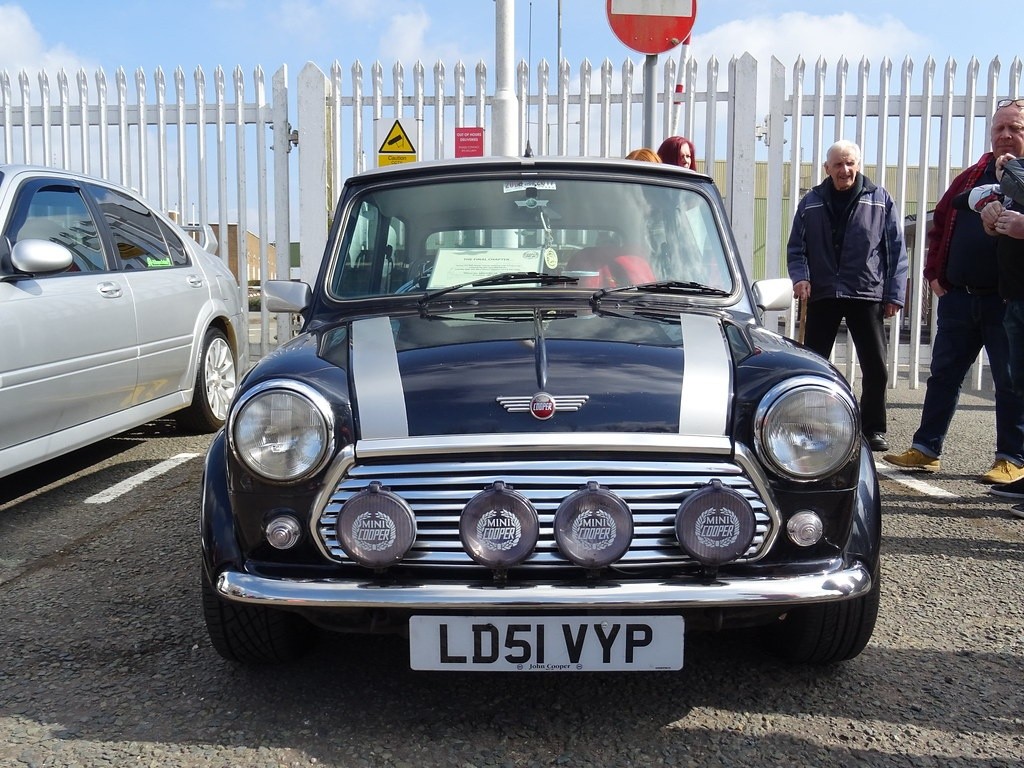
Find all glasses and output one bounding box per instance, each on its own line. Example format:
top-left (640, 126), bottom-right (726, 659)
top-left (997, 99), bottom-right (1024, 109)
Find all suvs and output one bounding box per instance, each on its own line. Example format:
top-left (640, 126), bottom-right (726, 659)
top-left (192, 157), bottom-right (890, 688)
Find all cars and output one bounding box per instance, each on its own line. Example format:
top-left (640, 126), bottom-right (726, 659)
top-left (0, 164), bottom-right (250, 496)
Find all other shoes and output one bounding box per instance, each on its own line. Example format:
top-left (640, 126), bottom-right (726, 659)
top-left (981, 457), bottom-right (1024, 483)
top-left (883, 448), bottom-right (940, 472)
top-left (991, 478), bottom-right (1024, 518)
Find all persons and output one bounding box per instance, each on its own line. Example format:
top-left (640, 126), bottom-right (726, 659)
top-left (785, 139), bottom-right (909, 451)
top-left (624, 148), bottom-right (662, 165)
top-left (882, 95), bottom-right (1024, 486)
top-left (656, 136), bottom-right (698, 172)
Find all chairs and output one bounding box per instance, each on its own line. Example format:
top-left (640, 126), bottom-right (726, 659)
top-left (16, 219), bottom-right (78, 243)
top-left (563, 246), bottom-right (658, 289)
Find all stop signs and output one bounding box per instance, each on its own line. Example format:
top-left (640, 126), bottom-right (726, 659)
top-left (605, 0), bottom-right (698, 57)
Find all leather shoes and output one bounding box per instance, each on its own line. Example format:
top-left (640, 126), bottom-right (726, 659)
top-left (866, 431), bottom-right (889, 451)
